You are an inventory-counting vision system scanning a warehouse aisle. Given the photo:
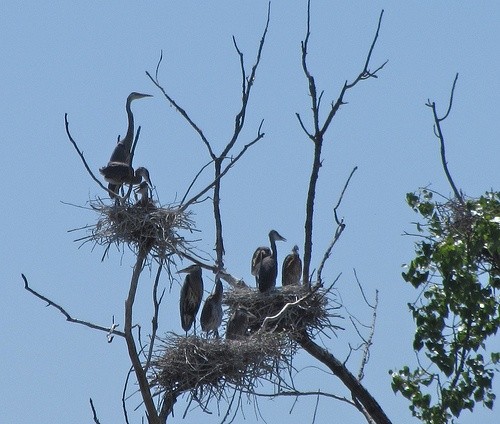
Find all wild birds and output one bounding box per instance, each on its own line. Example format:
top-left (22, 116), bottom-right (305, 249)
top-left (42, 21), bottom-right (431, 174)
top-left (200, 269), bottom-right (225, 338)
top-left (108, 92), bottom-right (154, 205)
top-left (252, 229), bottom-right (286, 291)
top-left (100, 163), bottom-right (153, 206)
top-left (282, 245), bottom-right (303, 287)
top-left (174, 264), bottom-right (204, 336)
top-left (227, 306), bottom-right (257, 340)
